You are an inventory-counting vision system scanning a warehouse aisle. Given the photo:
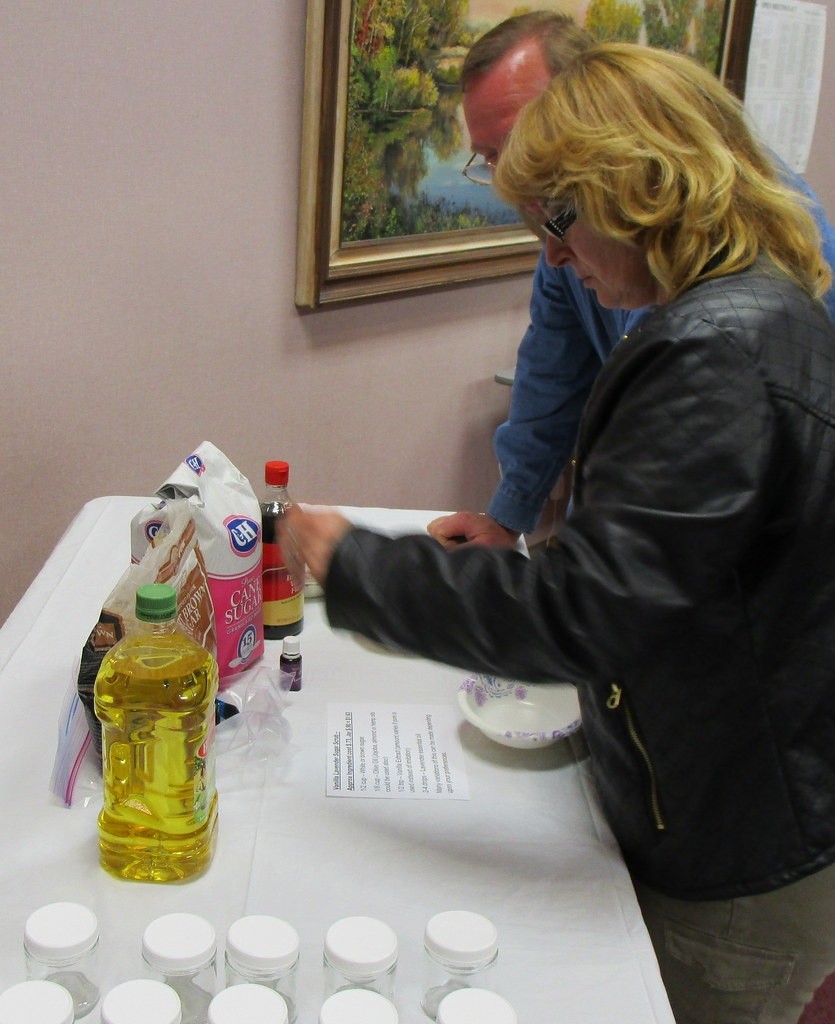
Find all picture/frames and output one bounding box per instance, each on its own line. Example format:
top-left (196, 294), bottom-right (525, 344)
top-left (293, 0), bottom-right (761, 315)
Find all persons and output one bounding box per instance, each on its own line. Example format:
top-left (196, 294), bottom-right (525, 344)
top-left (280, 10), bottom-right (835, 1024)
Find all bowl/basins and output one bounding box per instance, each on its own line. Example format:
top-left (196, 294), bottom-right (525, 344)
top-left (458, 669), bottom-right (580, 748)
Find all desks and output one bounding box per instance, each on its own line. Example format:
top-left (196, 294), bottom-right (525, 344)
top-left (0, 494), bottom-right (682, 1024)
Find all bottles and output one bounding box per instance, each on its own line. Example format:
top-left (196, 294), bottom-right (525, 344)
top-left (323, 917), bottom-right (397, 1003)
top-left (260, 460), bottom-right (306, 639)
top-left (24, 902), bottom-right (102, 1019)
top-left (95, 585), bottom-right (218, 881)
top-left (0, 980), bottom-right (75, 1024)
top-left (421, 911), bottom-right (499, 1018)
top-left (280, 636), bottom-right (302, 691)
top-left (207, 982), bottom-right (289, 1024)
top-left (436, 987), bottom-right (516, 1024)
top-left (224, 915), bottom-right (299, 1024)
top-left (142, 913), bottom-right (218, 1024)
top-left (318, 988), bottom-right (399, 1024)
top-left (102, 979), bottom-right (182, 1024)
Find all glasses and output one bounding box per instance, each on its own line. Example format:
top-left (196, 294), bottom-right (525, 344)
top-left (540, 207), bottom-right (577, 244)
top-left (462, 152), bottom-right (497, 187)
